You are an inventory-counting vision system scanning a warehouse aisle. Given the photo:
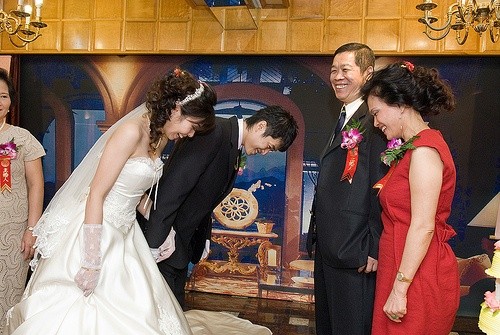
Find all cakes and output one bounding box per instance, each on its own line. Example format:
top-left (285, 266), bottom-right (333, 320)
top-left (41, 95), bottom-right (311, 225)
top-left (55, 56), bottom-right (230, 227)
top-left (478, 240), bottom-right (500, 335)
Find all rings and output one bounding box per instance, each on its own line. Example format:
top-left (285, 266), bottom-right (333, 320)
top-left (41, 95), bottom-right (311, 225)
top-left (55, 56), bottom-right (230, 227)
top-left (392, 315), bottom-right (397, 318)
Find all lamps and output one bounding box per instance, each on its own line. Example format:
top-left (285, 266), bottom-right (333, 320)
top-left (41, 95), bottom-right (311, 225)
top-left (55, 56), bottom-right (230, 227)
top-left (416, 0), bottom-right (500, 45)
top-left (0, 0), bottom-right (48, 48)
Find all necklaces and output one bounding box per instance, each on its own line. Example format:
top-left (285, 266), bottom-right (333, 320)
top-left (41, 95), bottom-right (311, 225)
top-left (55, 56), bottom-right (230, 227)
top-left (0, 118), bottom-right (6, 132)
top-left (146, 114), bottom-right (161, 149)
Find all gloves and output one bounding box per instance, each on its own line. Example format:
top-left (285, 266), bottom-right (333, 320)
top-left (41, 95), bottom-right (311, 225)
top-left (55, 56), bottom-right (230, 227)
top-left (149, 248), bottom-right (160, 260)
top-left (199, 240), bottom-right (209, 262)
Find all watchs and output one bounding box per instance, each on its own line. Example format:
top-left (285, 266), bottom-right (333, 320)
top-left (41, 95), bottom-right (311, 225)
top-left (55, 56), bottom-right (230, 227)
top-left (397, 272), bottom-right (412, 283)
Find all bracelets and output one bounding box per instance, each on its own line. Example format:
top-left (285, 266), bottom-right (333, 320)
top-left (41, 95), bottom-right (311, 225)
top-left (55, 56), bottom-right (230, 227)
top-left (26, 226), bottom-right (33, 230)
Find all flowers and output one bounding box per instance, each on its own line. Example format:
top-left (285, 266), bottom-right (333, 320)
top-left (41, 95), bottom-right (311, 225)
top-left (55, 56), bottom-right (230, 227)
top-left (379, 134), bottom-right (420, 167)
top-left (340, 118), bottom-right (367, 149)
top-left (0, 137), bottom-right (23, 160)
top-left (237, 153), bottom-right (248, 175)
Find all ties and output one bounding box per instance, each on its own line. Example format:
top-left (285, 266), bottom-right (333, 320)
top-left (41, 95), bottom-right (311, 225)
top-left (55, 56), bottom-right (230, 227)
top-left (331, 106), bottom-right (346, 145)
top-left (234, 148), bottom-right (241, 172)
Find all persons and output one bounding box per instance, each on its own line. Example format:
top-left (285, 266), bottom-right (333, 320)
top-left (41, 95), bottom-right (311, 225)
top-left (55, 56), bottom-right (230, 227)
top-left (135, 105), bottom-right (299, 311)
top-left (306, 42), bottom-right (404, 335)
top-left (3, 64), bottom-right (218, 335)
top-left (359, 60), bottom-right (461, 335)
top-left (0, 67), bottom-right (46, 335)
